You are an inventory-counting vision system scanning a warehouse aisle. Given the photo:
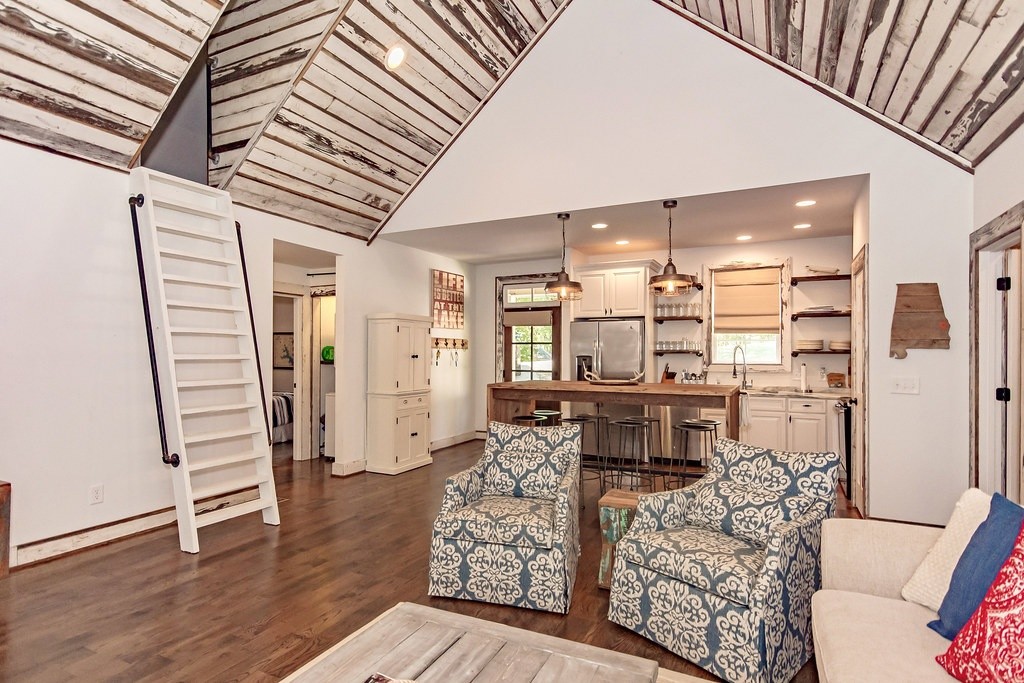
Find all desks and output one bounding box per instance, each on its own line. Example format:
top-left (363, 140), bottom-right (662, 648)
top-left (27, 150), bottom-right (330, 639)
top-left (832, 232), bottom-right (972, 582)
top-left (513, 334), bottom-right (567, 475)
top-left (486, 379), bottom-right (740, 441)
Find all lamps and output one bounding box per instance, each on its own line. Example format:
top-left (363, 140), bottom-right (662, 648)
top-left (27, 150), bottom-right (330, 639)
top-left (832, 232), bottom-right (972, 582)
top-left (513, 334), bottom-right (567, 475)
top-left (647, 199), bottom-right (694, 297)
top-left (544, 214), bottom-right (583, 303)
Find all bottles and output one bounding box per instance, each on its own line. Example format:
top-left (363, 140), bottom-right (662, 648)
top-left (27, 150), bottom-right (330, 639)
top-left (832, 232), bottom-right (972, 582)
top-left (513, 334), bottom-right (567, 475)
top-left (681, 362), bottom-right (708, 383)
top-left (656, 302), bottom-right (702, 317)
top-left (819, 367), bottom-right (829, 392)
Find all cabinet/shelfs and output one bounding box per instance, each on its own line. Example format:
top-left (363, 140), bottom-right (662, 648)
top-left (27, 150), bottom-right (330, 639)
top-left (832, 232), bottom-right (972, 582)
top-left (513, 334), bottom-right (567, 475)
top-left (653, 282), bottom-right (703, 357)
top-left (738, 394), bottom-right (827, 452)
top-left (365, 314), bottom-right (434, 395)
top-left (792, 272), bottom-right (851, 357)
top-left (325, 391), bottom-right (336, 462)
top-left (364, 394), bottom-right (434, 475)
top-left (571, 264), bottom-right (646, 318)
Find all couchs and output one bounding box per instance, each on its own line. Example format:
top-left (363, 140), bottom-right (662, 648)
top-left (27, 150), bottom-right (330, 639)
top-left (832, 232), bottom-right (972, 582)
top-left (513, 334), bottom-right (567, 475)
top-left (429, 421), bottom-right (582, 614)
top-left (811, 519), bottom-right (965, 683)
top-left (605, 436), bottom-right (840, 683)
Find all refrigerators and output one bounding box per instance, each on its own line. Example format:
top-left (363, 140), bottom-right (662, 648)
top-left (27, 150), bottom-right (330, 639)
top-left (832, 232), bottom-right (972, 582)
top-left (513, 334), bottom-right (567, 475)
top-left (570, 318), bottom-right (643, 461)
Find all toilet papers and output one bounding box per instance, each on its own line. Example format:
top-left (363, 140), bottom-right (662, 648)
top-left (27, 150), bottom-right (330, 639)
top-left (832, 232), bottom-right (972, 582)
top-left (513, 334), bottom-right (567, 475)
top-left (800, 366), bottom-right (807, 391)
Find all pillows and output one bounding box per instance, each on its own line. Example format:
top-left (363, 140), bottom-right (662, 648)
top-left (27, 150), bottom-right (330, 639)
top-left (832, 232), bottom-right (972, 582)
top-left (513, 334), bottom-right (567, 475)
top-left (934, 516), bottom-right (1024, 683)
top-left (901, 488), bottom-right (1024, 613)
top-left (927, 492), bottom-right (1024, 640)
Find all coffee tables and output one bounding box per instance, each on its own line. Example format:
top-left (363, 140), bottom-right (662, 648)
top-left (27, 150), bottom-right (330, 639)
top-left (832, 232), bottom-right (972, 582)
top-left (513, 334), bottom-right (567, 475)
top-left (277, 599), bottom-right (659, 683)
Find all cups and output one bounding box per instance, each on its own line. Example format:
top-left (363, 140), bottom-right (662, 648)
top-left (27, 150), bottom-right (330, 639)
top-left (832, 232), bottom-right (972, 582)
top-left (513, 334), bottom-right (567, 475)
top-left (657, 337), bottom-right (701, 351)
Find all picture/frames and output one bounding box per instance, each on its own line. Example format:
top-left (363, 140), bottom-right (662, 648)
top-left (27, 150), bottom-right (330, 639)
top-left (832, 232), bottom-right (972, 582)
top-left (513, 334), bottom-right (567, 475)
top-left (273, 331), bottom-right (294, 370)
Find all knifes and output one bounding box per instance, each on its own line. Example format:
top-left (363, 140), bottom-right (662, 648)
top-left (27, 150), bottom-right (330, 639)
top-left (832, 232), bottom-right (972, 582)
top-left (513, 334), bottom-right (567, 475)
top-left (665, 363), bottom-right (677, 380)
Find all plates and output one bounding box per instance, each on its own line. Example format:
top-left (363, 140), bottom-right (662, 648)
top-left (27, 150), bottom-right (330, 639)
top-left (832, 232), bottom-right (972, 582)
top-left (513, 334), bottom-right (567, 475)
top-left (795, 338), bottom-right (851, 350)
top-left (798, 305), bottom-right (841, 313)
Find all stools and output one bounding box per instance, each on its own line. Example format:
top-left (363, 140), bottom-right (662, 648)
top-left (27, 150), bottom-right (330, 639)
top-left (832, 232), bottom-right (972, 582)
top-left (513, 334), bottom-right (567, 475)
top-left (512, 415), bottom-right (547, 428)
top-left (557, 417), bottom-right (602, 510)
top-left (677, 419), bottom-right (721, 488)
top-left (529, 410), bottom-right (563, 427)
top-left (666, 424), bottom-right (716, 491)
top-left (575, 413), bottom-right (615, 489)
top-left (619, 416), bottom-right (667, 492)
top-left (603, 420), bottom-right (654, 494)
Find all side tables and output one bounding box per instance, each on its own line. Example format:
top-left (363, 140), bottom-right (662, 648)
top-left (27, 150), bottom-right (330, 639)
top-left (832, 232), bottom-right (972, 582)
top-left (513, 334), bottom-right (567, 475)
top-left (597, 490), bottom-right (651, 589)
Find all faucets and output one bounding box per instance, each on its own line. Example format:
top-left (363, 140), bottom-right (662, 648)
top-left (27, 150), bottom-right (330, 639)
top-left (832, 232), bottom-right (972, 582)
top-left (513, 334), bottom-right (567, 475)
top-left (732, 344), bottom-right (753, 390)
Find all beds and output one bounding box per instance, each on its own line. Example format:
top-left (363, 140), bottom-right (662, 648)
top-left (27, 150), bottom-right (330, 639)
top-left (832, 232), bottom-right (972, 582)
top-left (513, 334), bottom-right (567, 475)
top-left (271, 392), bottom-right (297, 443)
